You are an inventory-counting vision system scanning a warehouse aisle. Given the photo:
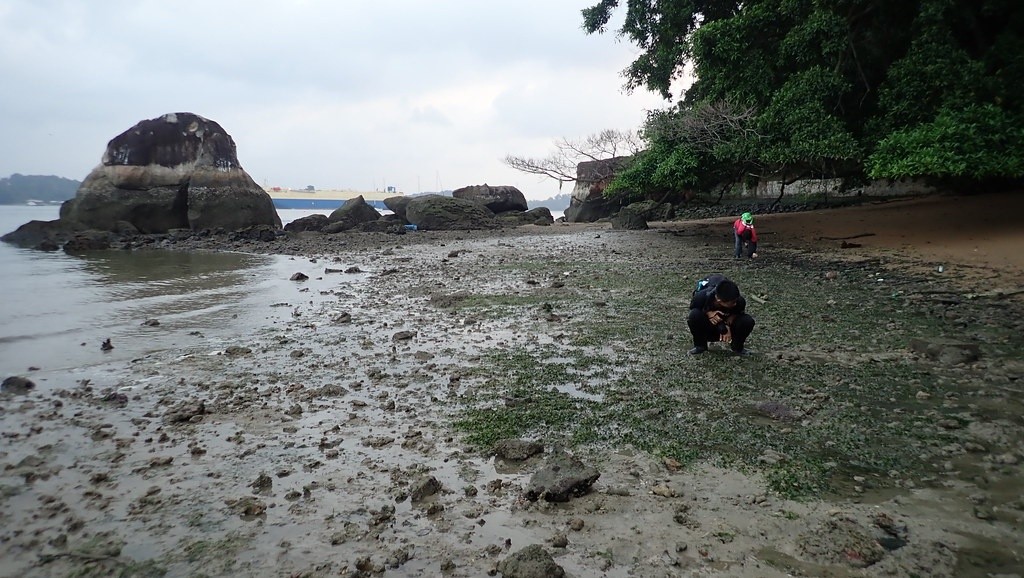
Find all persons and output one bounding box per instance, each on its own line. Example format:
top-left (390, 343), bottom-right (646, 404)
top-left (733, 212), bottom-right (758, 261)
top-left (687, 279), bottom-right (755, 356)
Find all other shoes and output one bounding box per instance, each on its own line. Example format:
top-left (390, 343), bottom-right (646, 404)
top-left (729, 348), bottom-right (751, 357)
top-left (749, 256), bottom-right (754, 262)
top-left (688, 346), bottom-right (708, 354)
top-left (735, 254), bottom-right (742, 260)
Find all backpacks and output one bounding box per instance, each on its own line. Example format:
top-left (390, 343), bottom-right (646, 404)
top-left (691, 271), bottom-right (727, 298)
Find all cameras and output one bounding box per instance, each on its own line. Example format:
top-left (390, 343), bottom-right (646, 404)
top-left (715, 313), bottom-right (729, 335)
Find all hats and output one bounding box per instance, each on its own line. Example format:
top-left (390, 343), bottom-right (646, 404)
top-left (741, 213), bottom-right (753, 225)
